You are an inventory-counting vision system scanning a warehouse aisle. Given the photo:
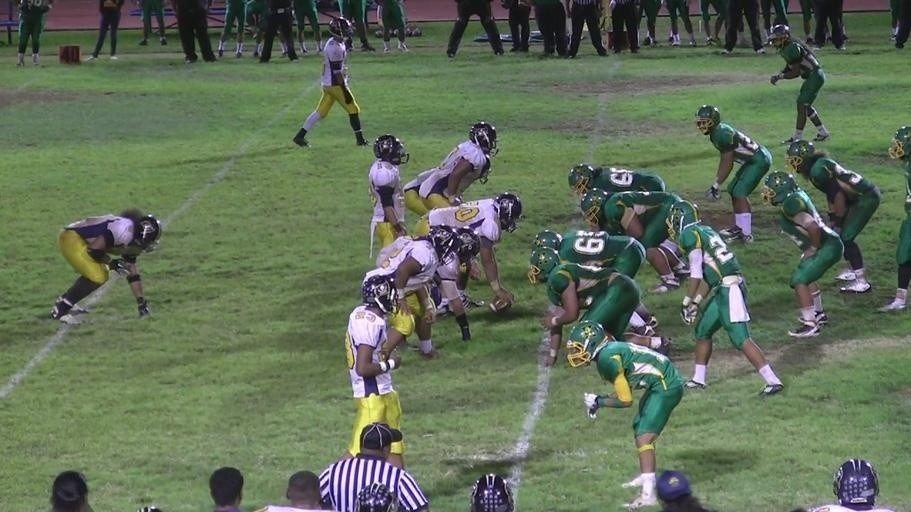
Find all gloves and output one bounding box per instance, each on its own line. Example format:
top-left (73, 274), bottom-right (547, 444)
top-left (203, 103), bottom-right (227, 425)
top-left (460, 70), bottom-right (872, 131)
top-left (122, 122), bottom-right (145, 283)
top-left (680, 294), bottom-right (703, 325)
top-left (109, 259), bottom-right (127, 273)
top-left (706, 183), bottom-right (720, 202)
top-left (771, 74), bottom-right (783, 85)
top-left (583, 392), bottom-right (599, 419)
top-left (138, 301), bottom-right (147, 315)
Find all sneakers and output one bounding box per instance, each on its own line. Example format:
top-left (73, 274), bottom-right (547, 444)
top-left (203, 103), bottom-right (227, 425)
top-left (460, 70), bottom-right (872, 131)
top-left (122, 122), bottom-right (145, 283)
top-left (293, 136), bottom-right (308, 146)
top-left (436, 295), bottom-right (484, 319)
top-left (813, 132), bottom-right (829, 141)
top-left (622, 477), bottom-right (658, 507)
top-left (835, 269), bottom-right (872, 293)
top-left (634, 314), bottom-right (670, 356)
top-left (649, 265), bottom-right (690, 293)
top-left (760, 384), bottom-right (783, 394)
top-left (788, 309), bottom-right (827, 338)
top-left (720, 225), bottom-right (753, 244)
top-left (683, 379), bottom-right (705, 388)
top-left (51, 304), bottom-right (88, 323)
top-left (878, 302), bottom-right (907, 311)
top-left (781, 136), bottom-right (800, 144)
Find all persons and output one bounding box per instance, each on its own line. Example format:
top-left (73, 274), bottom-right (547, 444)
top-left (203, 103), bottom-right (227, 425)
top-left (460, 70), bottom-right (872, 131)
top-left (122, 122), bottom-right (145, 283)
top-left (656, 469), bottom-right (705, 510)
top-left (567, 319), bottom-right (685, 509)
top-left (49, 211), bottom-right (164, 325)
top-left (319, 422), bottom-right (431, 510)
top-left (208, 465), bottom-right (245, 511)
top-left (447, 1), bottom-right (849, 60)
top-left (889, 1), bottom-right (911, 51)
top-left (344, 119), bottom-right (523, 511)
top-left (528, 163), bottom-right (783, 397)
top-left (877, 125), bottom-right (911, 312)
top-left (291, 17), bottom-right (373, 148)
top-left (784, 139), bottom-right (882, 296)
top-left (798, 458), bottom-right (902, 510)
top-left (252, 472), bottom-right (326, 510)
top-left (695, 105), bottom-right (775, 243)
top-left (87, 0), bottom-right (409, 62)
top-left (15, 1), bottom-right (55, 66)
top-left (759, 171), bottom-right (845, 338)
top-left (50, 470), bottom-right (94, 511)
top-left (768, 24), bottom-right (832, 145)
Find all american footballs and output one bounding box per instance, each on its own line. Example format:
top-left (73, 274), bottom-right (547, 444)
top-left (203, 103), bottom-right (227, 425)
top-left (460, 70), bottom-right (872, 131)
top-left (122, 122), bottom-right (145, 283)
top-left (490, 296), bottom-right (513, 314)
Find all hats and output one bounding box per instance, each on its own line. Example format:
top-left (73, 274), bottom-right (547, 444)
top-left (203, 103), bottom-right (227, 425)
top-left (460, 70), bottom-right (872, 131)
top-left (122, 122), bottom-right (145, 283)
top-left (657, 471), bottom-right (691, 499)
top-left (359, 422), bottom-right (402, 449)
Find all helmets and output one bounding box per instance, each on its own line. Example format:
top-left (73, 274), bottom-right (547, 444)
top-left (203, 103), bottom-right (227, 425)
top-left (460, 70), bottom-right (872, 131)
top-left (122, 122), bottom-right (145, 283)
top-left (356, 483), bottom-right (398, 512)
top-left (769, 25), bottom-right (790, 47)
top-left (665, 199), bottom-right (700, 240)
top-left (833, 458), bottom-right (879, 503)
top-left (373, 135), bottom-right (409, 165)
top-left (527, 230), bottom-right (608, 367)
top-left (696, 105), bottom-right (720, 136)
top-left (328, 17), bottom-right (353, 41)
top-left (568, 164), bottom-right (608, 226)
top-left (136, 217), bottom-right (159, 250)
top-left (469, 122), bottom-right (499, 157)
top-left (470, 474), bottom-right (514, 512)
top-left (762, 140), bottom-right (814, 206)
top-left (363, 193), bottom-right (522, 314)
top-left (888, 126), bottom-right (911, 160)
